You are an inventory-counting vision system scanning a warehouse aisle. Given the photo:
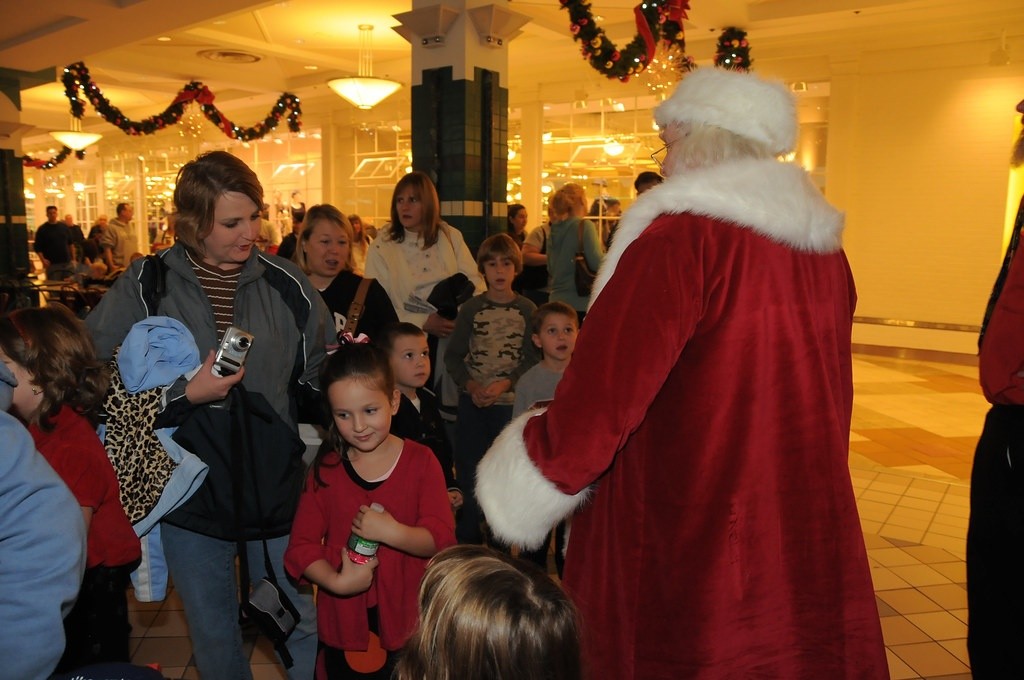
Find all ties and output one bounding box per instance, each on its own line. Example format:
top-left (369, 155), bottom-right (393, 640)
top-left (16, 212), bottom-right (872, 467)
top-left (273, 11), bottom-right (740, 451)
top-left (978, 195), bottom-right (1024, 354)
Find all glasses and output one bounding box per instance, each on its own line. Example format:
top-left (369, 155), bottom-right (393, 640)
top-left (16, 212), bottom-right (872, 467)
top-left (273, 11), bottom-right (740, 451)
top-left (651, 130), bottom-right (689, 173)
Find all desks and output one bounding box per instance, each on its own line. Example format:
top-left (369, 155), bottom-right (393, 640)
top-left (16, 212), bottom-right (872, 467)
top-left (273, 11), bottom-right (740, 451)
top-left (5, 278), bottom-right (70, 290)
top-left (33, 285), bottom-right (109, 294)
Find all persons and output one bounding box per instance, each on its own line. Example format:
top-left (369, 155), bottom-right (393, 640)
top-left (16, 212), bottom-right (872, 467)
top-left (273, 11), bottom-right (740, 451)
top-left (442, 171), bottom-right (670, 587)
top-left (287, 192), bottom-right (305, 234)
top-left (478, 69), bottom-right (889, 680)
top-left (969, 95), bottom-right (1024, 678)
top-left (390, 544), bottom-right (583, 680)
top-left (79, 150), bottom-right (488, 680)
top-left (0, 306), bottom-right (143, 680)
top-left (33, 203), bottom-right (139, 283)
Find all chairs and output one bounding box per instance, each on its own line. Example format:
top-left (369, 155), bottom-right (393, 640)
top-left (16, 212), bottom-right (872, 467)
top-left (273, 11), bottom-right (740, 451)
top-left (0, 269), bottom-right (118, 320)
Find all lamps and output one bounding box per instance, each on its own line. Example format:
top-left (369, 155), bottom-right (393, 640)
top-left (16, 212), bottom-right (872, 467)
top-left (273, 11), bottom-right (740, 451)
top-left (328, 25), bottom-right (402, 110)
top-left (49, 99), bottom-right (103, 152)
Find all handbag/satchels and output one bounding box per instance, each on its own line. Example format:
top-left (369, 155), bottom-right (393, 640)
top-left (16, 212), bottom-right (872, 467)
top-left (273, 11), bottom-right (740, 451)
top-left (574, 219), bottom-right (598, 296)
top-left (246, 575), bottom-right (302, 650)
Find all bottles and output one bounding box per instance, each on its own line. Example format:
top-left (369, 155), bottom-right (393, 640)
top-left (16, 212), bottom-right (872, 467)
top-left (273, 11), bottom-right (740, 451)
top-left (336, 502), bottom-right (384, 572)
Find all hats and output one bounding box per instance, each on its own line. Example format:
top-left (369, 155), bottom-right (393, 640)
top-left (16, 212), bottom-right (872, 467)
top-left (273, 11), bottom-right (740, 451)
top-left (653, 64), bottom-right (797, 158)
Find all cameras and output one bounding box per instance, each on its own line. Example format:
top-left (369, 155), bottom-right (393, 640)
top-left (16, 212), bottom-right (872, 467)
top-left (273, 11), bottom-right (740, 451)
top-left (211, 327), bottom-right (254, 377)
